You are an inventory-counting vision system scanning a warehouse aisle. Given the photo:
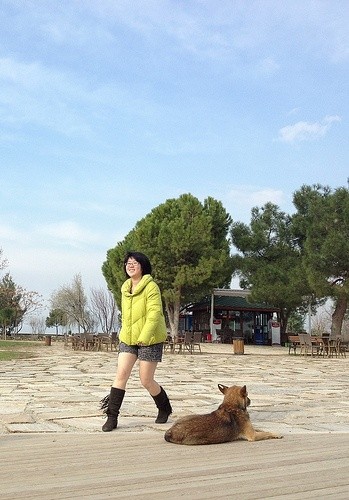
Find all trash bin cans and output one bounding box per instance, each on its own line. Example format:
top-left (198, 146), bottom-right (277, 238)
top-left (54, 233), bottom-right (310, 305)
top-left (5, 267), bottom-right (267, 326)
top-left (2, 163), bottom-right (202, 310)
top-left (232, 336), bottom-right (244, 355)
top-left (44, 336), bottom-right (51, 346)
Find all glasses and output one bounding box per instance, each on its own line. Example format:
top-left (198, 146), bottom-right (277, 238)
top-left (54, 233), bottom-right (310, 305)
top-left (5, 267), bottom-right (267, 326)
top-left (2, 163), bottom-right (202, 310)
top-left (125, 262), bottom-right (140, 266)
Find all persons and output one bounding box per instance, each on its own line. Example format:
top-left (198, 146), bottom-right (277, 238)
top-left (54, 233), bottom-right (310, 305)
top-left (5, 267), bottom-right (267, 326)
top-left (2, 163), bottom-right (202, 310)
top-left (99, 251), bottom-right (173, 432)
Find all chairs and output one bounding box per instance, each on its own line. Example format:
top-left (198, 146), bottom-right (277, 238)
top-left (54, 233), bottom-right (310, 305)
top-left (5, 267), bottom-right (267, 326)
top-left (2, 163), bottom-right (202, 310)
top-left (288, 333), bottom-right (347, 359)
top-left (64, 331), bottom-right (202, 354)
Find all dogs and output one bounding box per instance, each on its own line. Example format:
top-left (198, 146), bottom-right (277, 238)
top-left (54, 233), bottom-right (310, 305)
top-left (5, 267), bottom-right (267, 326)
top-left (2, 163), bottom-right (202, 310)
top-left (164, 384), bottom-right (284, 446)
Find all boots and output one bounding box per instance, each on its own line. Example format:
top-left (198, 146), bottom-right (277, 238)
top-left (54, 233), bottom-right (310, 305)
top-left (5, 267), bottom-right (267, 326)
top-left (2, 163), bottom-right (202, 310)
top-left (99, 387), bottom-right (125, 432)
top-left (150, 386), bottom-right (172, 423)
